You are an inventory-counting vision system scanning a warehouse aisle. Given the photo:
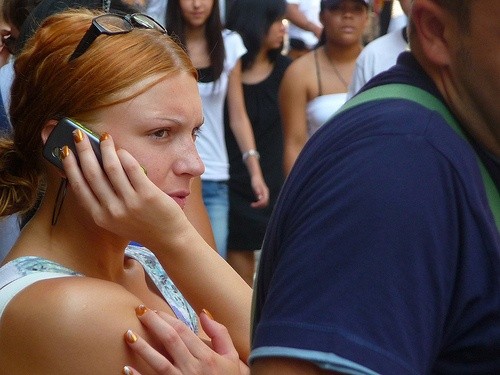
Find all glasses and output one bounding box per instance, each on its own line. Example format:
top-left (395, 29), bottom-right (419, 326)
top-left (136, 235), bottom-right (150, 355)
top-left (68, 12), bottom-right (167, 61)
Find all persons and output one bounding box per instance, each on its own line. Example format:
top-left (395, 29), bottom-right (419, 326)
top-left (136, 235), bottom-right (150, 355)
top-left (0, 0), bottom-right (413, 375)
top-left (246, 0), bottom-right (500, 375)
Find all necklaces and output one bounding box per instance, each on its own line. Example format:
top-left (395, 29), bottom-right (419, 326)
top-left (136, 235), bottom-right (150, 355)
top-left (324, 46), bottom-right (348, 88)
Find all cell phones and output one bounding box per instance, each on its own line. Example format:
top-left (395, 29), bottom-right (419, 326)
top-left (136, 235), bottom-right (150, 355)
top-left (43, 117), bottom-right (147, 177)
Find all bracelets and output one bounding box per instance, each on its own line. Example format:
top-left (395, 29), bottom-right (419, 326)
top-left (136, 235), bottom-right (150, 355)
top-left (241, 150), bottom-right (260, 160)
